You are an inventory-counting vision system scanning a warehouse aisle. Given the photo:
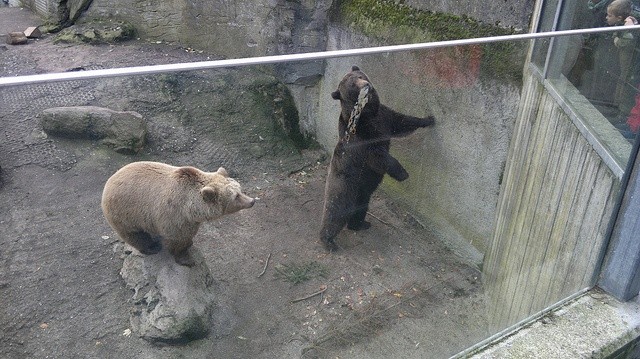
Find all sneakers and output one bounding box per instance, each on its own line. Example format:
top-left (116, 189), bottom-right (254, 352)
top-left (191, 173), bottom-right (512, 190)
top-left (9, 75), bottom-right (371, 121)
top-left (617, 123), bottom-right (630, 131)
top-left (621, 129), bottom-right (635, 139)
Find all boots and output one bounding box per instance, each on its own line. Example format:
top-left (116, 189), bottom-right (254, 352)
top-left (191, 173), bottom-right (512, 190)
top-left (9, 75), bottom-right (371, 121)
top-left (570, 59), bottom-right (586, 86)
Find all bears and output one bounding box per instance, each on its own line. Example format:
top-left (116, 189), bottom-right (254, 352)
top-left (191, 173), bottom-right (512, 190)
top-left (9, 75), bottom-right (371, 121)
top-left (318, 65), bottom-right (436, 252)
top-left (101, 160), bottom-right (255, 267)
